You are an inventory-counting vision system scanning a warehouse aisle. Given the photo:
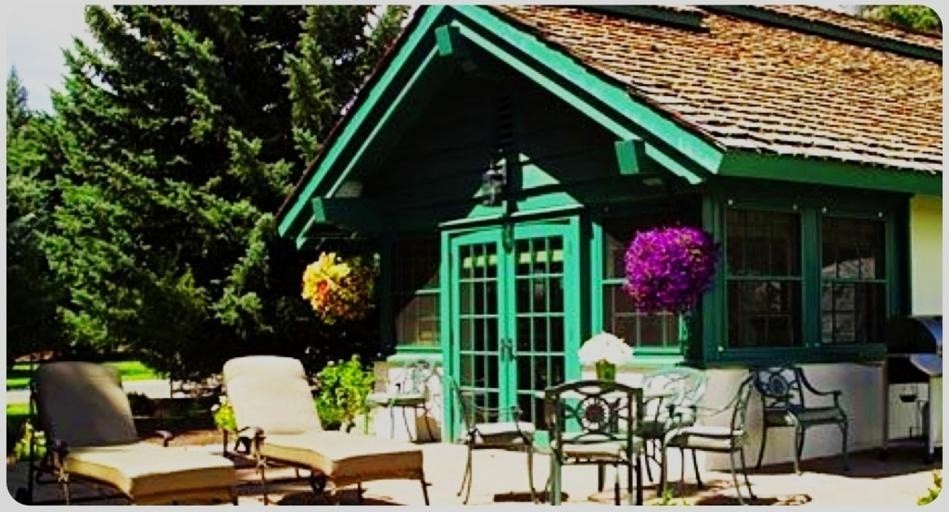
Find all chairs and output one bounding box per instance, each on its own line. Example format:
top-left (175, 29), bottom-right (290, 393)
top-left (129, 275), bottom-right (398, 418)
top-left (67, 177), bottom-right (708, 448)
top-left (223, 354), bottom-right (432, 505)
top-left (622, 365), bottom-right (757, 504)
top-left (543, 380), bottom-right (645, 505)
top-left (446, 375), bottom-right (537, 504)
top-left (368, 356), bottom-right (435, 441)
top-left (749, 358), bottom-right (850, 475)
top-left (27, 359), bottom-right (238, 505)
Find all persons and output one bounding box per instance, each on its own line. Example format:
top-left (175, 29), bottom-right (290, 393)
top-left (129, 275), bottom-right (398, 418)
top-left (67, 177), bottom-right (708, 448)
top-left (736, 287), bottom-right (780, 347)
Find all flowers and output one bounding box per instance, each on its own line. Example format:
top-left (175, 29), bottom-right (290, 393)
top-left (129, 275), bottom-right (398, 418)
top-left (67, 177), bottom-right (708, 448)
top-left (301, 249), bottom-right (380, 325)
top-left (578, 331), bottom-right (634, 367)
top-left (625, 226), bottom-right (724, 316)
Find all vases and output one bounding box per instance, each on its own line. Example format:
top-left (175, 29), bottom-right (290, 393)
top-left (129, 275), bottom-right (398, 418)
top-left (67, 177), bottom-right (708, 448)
top-left (596, 360), bottom-right (615, 382)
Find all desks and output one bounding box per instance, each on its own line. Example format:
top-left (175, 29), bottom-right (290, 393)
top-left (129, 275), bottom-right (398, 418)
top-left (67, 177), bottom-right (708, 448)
top-left (517, 388), bottom-right (679, 504)
